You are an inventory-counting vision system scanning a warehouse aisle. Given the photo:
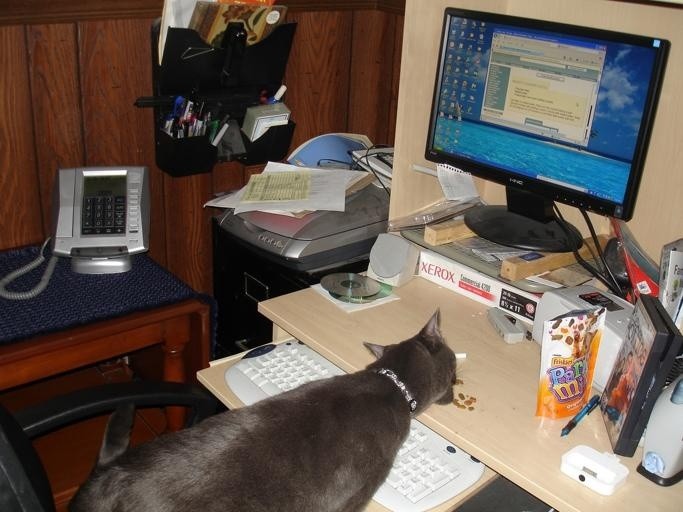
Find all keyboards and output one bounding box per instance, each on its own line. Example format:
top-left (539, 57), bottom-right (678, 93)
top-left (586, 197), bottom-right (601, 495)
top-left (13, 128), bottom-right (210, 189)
top-left (225, 338), bottom-right (486, 512)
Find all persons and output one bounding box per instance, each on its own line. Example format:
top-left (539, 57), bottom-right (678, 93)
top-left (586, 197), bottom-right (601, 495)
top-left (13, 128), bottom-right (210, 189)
top-left (609, 351), bottom-right (637, 420)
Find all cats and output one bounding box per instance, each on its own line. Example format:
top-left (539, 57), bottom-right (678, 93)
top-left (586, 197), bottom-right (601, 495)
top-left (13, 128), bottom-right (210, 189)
top-left (67, 307), bottom-right (463, 512)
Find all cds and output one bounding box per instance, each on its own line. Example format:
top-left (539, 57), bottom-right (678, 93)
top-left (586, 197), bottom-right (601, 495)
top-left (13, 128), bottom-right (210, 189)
top-left (320, 273), bottom-right (393, 304)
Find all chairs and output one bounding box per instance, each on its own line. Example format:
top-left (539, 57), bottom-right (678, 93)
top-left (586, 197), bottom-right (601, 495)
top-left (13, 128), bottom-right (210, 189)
top-left (0, 380), bottom-right (212, 512)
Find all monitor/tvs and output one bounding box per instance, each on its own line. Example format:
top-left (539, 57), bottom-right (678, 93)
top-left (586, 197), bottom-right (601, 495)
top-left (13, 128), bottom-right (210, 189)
top-left (425, 7), bottom-right (671, 253)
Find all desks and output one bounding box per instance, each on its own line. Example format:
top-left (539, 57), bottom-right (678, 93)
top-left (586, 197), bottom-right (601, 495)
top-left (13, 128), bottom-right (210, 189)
top-left (0, 243), bottom-right (200, 472)
top-left (198, 267), bottom-right (683, 512)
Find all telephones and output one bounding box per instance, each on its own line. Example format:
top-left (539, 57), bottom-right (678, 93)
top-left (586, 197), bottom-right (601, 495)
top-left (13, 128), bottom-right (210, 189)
top-left (50, 165), bottom-right (151, 274)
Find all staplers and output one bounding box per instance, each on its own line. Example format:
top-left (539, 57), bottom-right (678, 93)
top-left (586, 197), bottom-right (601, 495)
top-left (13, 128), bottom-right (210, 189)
top-left (487, 306), bottom-right (533, 344)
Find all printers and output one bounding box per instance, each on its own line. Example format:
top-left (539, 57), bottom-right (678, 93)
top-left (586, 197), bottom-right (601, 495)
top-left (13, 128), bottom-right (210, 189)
top-left (210, 183), bottom-right (391, 360)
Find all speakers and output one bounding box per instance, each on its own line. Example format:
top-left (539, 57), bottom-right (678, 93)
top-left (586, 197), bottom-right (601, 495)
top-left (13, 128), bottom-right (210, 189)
top-left (367, 233), bottom-right (420, 288)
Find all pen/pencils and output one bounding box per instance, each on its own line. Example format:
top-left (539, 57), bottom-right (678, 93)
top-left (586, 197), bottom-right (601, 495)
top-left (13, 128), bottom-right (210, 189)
top-left (161, 96), bottom-right (232, 147)
top-left (560, 395), bottom-right (601, 438)
